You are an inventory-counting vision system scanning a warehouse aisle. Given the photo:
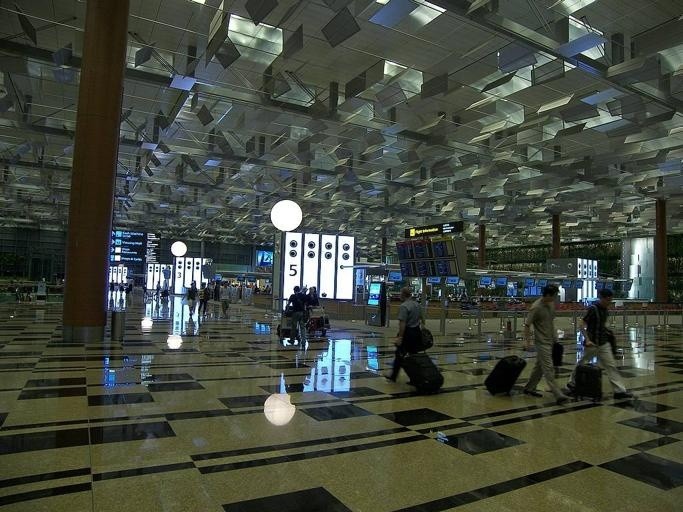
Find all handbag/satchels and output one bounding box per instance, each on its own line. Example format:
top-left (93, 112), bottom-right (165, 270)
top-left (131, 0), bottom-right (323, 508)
top-left (420, 328), bottom-right (433, 351)
top-left (607, 334), bottom-right (617, 355)
top-left (552, 343), bottom-right (564, 367)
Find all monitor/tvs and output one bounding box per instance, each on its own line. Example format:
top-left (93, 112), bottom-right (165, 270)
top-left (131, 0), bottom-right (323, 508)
top-left (495, 276), bottom-right (548, 297)
top-left (237, 276), bottom-right (245, 281)
top-left (215, 274), bottom-right (221, 281)
top-left (250, 248), bottom-right (274, 267)
top-left (595, 282), bottom-right (604, 289)
top-left (247, 275), bottom-right (256, 282)
top-left (562, 280), bottom-right (572, 289)
top-left (574, 280), bottom-right (583, 289)
top-left (387, 272), bottom-right (403, 283)
top-left (605, 282), bottom-right (613, 289)
top-left (480, 275), bottom-right (492, 285)
top-left (396, 237), bottom-right (467, 284)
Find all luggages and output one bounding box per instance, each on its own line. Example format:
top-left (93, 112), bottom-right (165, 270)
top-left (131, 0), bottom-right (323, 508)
top-left (306, 305), bottom-right (330, 331)
top-left (574, 345), bottom-right (602, 398)
top-left (485, 355), bottom-right (529, 395)
top-left (392, 341), bottom-right (444, 393)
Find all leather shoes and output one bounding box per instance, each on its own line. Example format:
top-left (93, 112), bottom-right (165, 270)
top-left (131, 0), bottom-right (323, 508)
top-left (287, 340), bottom-right (293, 345)
top-left (613, 392), bottom-right (633, 399)
top-left (383, 372), bottom-right (395, 382)
top-left (302, 343), bottom-right (306, 345)
top-left (556, 397), bottom-right (569, 405)
top-left (524, 389), bottom-right (543, 397)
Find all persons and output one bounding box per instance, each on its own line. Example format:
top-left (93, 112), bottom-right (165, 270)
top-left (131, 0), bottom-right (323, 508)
top-left (126, 276), bottom-right (133, 291)
top-left (196, 282), bottom-right (208, 318)
top-left (284, 285), bottom-right (307, 346)
top-left (163, 279), bottom-right (167, 291)
top-left (519, 284), bottom-right (570, 405)
top-left (566, 288), bottom-right (634, 400)
top-left (109, 279), bottom-right (115, 298)
top-left (15, 286), bottom-right (21, 304)
top-left (218, 281), bottom-right (232, 319)
top-left (184, 282), bottom-right (197, 316)
top-left (302, 287), bottom-right (318, 329)
top-left (155, 280), bottom-right (161, 300)
top-left (118, 279), bottom-right (124, 298)
top-left (382, 286), bottom-right (421, 387)
top-left (207, 277), bottom-right (272, 301)
